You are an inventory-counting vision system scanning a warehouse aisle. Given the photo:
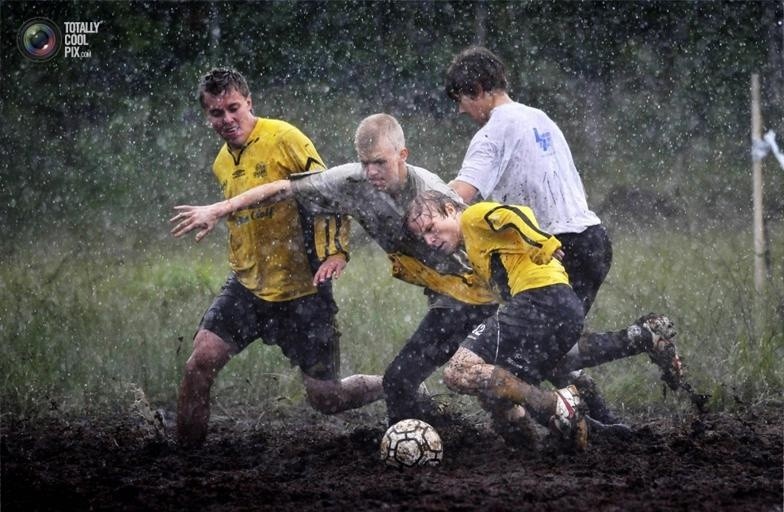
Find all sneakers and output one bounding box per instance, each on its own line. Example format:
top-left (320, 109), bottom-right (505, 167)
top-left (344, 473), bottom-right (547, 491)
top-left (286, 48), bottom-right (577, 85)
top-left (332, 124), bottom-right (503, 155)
top-left (635, 313), bottom-right (683, 390)
top-left (548, 385), bottom-right (588, 451)
top-left (416, 381), bottom-right (449, 416)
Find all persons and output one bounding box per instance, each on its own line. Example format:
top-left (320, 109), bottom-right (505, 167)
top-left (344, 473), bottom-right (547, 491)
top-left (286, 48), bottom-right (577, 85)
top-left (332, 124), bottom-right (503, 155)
top-left (173, 69), bottom-right (429, 441)
top-left (165, 113), bottom-right (501, 431)
top-left (442, 45), bottom-right (684, 428)
top-left (400, 190), bottom-right (590, 452)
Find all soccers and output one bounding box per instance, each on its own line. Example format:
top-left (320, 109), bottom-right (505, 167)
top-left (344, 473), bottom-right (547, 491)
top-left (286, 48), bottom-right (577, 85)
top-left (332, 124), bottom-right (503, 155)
top-left (381, 419), bottom-right (442, 469)
